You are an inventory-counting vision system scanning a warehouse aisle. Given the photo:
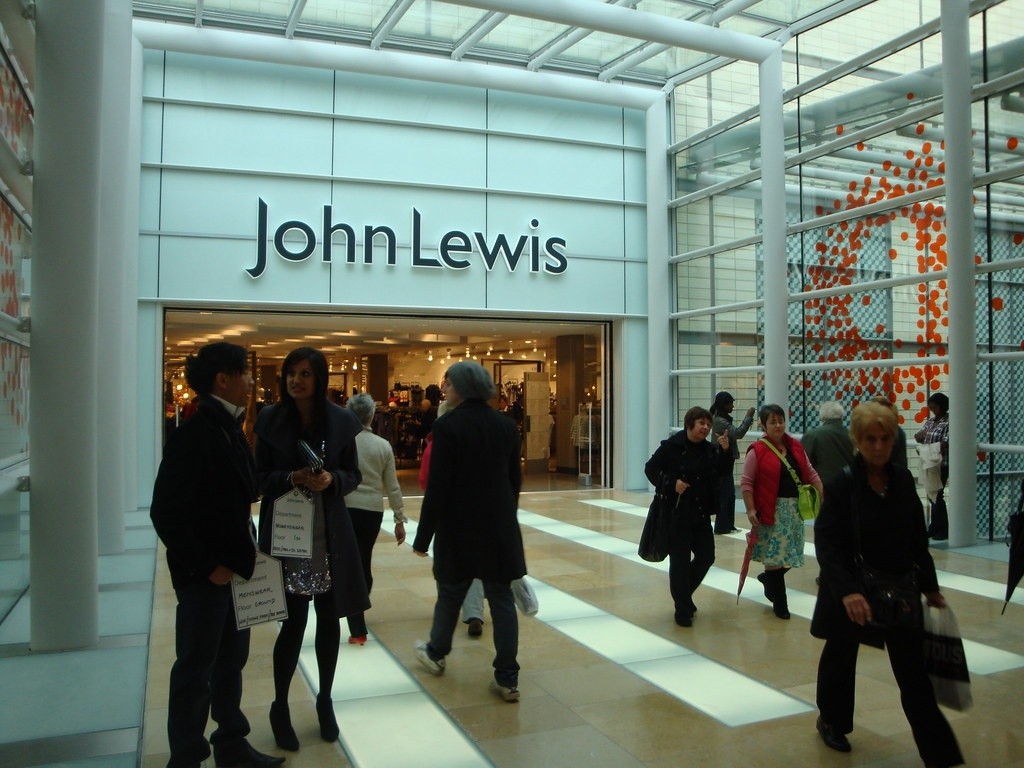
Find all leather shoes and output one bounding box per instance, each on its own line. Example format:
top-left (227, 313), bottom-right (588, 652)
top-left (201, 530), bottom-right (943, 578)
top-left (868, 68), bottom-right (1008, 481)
top-left (816, 714), bottom-right (851, 753)
top-left (213, 737), bottom-right (287, 768)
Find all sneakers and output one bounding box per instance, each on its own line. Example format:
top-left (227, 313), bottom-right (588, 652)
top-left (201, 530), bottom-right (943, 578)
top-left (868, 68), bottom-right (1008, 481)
top-left (489, 677), bottom-right (520, 702)
top-left (413, 639), bottom-right (446, 675)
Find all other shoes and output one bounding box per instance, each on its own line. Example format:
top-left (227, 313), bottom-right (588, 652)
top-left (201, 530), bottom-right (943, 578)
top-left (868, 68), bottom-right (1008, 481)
top-left (715, 527), bottom-right (742, 534)
top-left (469, 618), bottom-right (482, 636)
top-left (673, 595), bottom-right (697, 627)
top-left (349, 633), bottom-right (367, 646)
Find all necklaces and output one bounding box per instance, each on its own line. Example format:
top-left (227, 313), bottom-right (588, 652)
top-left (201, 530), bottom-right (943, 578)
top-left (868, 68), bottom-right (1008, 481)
top-left (878, 477), bottom-right (889, 497)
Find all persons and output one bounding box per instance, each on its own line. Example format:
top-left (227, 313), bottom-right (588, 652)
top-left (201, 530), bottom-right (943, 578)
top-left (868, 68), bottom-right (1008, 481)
top-left (344, 393), bottom-right (409, 648)
top-left (799, 401), bottom-right (856, 587)
top-left (252, 346), bottom-right (367, 753)
top-left (148, 341), bottom-right (288, 768)
top-left (914, 393), bottom-right (949, 541)
top-left (412, 360), bottom-right (539, 702)
top-left (638, 407), bottom-right (729, 627)
top-left (868, 396), bottom-right (908, 469)
top-left (741, 404), bottom-right (824, 620)
top-left (709, 391), bottom-right (756, 535)
top-left (809, 401), bottom-right (967, 768)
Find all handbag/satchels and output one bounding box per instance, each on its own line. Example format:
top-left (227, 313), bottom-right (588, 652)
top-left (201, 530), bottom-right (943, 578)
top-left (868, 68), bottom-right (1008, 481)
top-left (510, 576), bottom-right (539, 618)
top-left (797, 483), bottom-right (821, 521)
top-left (928, 488), bottom-right (949, 540)
top-left (637, 470), bottom-right (675, 562)
top-left (282, 488), bottom-right (333, 595)
top-left (918, 597), bottom-right (973, 713)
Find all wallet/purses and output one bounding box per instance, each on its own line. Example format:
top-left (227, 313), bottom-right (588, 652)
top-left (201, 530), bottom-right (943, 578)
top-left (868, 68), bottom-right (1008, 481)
top-left (292, 438), bottom-right (324, 499)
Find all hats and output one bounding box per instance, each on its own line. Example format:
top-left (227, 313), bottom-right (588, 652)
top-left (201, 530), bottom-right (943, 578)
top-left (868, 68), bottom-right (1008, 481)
top-left (716, 391), bottom-right (735, 402)
top-left (447, 361), bottom-right (494, 402)
top-left (426, 384), bottom-right (443, 398)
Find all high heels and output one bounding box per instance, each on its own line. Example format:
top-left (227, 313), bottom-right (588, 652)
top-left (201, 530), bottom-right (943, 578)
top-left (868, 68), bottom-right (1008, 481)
top-left (316, 693), bottom-right (340, 742)
top-left (269, 700), bottom-right (299, 751)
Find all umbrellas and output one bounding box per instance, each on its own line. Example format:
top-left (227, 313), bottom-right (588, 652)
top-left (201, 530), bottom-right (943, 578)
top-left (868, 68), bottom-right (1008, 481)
top-left (999, 478), bottom-right (1024, 616)
top-left (736, 511), bottom-right (762, 606)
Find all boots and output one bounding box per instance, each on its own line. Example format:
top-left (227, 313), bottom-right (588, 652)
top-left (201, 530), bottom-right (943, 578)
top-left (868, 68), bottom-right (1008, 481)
top-left (757, 567), bottom-right (791, 619)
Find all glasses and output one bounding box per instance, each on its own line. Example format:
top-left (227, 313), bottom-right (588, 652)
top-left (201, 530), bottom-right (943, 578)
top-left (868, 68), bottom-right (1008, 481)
top-left (442, 381), bottom-right (448, 384)
